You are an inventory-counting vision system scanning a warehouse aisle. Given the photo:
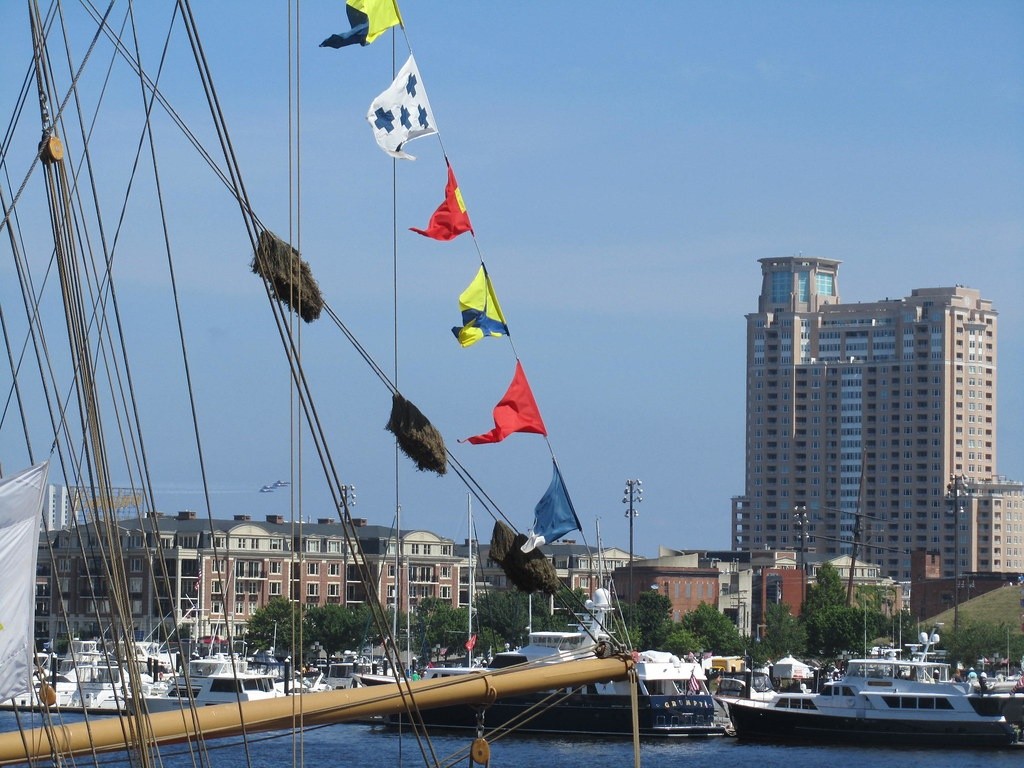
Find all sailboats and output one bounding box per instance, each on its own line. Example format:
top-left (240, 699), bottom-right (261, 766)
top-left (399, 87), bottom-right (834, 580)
top-left (0, 2), bottom-right (642, 768)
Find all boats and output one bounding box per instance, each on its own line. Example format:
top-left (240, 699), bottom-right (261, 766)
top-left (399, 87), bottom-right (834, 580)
top-left (353, 585), bottom-right (726, 741)
top-left (3, 632), bottom-right (393, 719)
top-left (712, 632), bottom-right (1013, 751)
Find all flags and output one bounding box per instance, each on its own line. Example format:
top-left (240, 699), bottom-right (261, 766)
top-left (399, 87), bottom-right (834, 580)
top-left (320, 0), bottom-right (580, 554)
top-left (466, 635), bottom-right (476, 652)
top-left (688, 675), bottom-right (700, 693)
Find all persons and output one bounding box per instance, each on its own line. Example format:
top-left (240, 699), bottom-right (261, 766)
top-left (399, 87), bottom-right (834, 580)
top-left (950, 666), bottom-right (987, 683)
top-left (412, 669), bottom-right (419, 680)
